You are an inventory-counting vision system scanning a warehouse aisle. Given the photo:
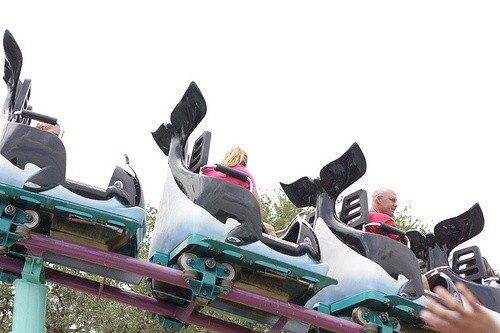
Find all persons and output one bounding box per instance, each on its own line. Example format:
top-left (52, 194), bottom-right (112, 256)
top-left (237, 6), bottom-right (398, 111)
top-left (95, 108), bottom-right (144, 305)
top-left (36, 120), bottom-right (61, 138)
top-left (465, 255), bottom-right (499, 287)
top-left (419, 281), bottom-right (499, 333)
top-left (203, 147), bottom-right (278, 239)
top-left (365, 185), bottom-right (431, 291)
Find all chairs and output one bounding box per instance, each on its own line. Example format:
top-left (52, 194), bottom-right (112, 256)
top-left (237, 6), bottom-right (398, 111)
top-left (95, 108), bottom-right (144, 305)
top-left (451, 245), bottom-right (489, 284)
top-left (339, 190), bottom-right (372, 233)
top-left (187, 131), bottom-right (213, 174)
